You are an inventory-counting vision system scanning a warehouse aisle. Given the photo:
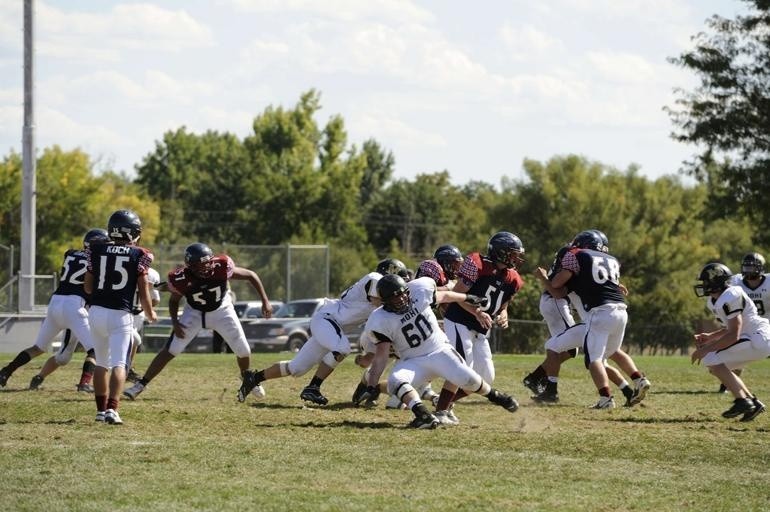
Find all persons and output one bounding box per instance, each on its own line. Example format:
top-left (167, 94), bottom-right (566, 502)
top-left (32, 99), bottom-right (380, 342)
top-left (86, 208), bottom-right (162, 425)
top-left (356, 272), bottom-right (519, 438)
top-left (692, 251), bottom-right (769, 423)
top-left (0, 227), bottom-right (274, 401)
top-left (237, 232), bottom-right (524, 421)
top-left (523, 229), bottom-right (652, 409)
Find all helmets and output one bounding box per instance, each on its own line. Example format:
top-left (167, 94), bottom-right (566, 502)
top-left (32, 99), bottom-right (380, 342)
top-left (486, 231), bottom-right (528, 272)
top-left (108, 210), bottom-right (142, 245)
top-left (83, 229), bottom-right (107, 250)
top-left (184, 243), bottom-right (215, 284)
top-left (739, 251), bottom-right (766, 279)
top-left (693, 262), bottom-right (733, 297)
top-left (570, 229), bottom-right (610, 253)
top-left (376, 245), bottom-right (465, 315)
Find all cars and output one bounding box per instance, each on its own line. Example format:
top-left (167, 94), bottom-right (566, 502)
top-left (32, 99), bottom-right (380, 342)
top-left (221, 302), bottom-right (282, 351)
top-left (245, 298), bottom-right (328, 351)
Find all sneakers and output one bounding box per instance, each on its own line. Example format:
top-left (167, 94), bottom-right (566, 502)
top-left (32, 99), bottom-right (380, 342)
top-left (300, 384), bottom-right (328, 406)
top-left (588, 396), bottom-right (615, 408)
top-left (522, 373), bottom-right (560, 407)
top-left (30, 374), bottom-right (42, 390)
top-left (722, 394), bottom-right (766, 422)
top-left (720, 383), bottom-right (727, 392)
top-left (124, 367), bottom-right (142, 382)
top-left (351, 382), bottom-right (460, 430)
top-left (95, 407), bottom-right (123, 426)
top-left (623, 377), bottom-right (650, 406)
top-left (76, 382), bottom-right (94, 394)
top-left (488, 388), bottom-right (519, 413)
top-left (123, 383), bottom-right (145, 399)
top-left (0, 365), bottom-right (10, 388)
top-left (236, 369), bottom-right (266, 403)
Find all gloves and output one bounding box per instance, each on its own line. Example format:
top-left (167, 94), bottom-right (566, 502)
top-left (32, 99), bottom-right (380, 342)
top-left (464, 294), bottom-right (487, 308)
